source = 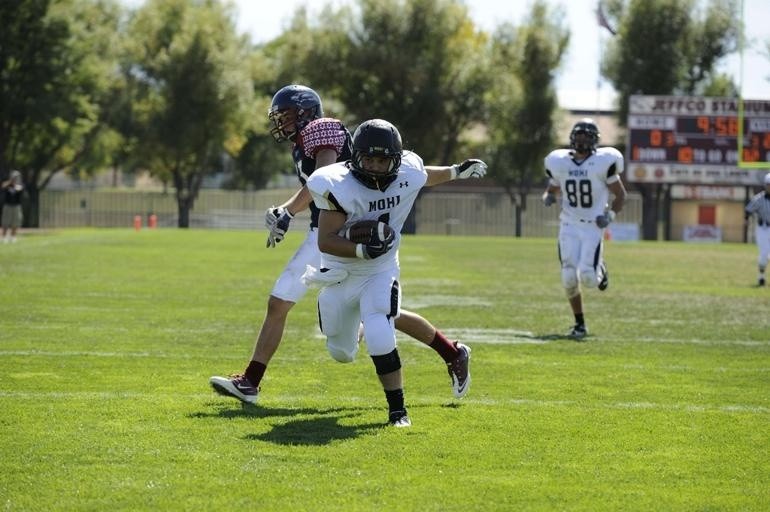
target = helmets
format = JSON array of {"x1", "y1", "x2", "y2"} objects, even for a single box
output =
[
  {"x1": 569, "y1": 118, "x2": 600, "y2": 156},
  {"x1": 345, "y1": 118, "x2": 403, "y2": 190},
  {"x1": 269, "y1": 85, "x2": 323, "y2": 143}
]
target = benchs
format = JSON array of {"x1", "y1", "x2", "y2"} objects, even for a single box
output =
[{"x1": 684, "y1": 225, "x2": 722, "y2": 241}]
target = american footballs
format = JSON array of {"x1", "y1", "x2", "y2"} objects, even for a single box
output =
[{"x1": 344, "y1": 220, "x2": 394, "y2": 245}]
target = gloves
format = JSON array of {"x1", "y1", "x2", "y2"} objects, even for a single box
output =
[
  {"x1": 594, "y1": 211, "x2": 612, "y2": 226},
  {"x1": 265, "y1": 206, "x2": 284, "y2": 227},
  {"x1": 453, "y1": 158, "x2": 488, "y2": 180},
  {"x1": 356, "y1": 241, "x2": 388, "y2": 261},
  {"x1": 541, "y1": 191, "x2": 557, "y2": 208},
  {"x1": 267, "y1": 208, "x2": 294, "y2": 248}
]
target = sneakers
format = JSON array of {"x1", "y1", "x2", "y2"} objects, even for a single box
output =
[
  {"x1": 389, "y1": 407, "x2": 412, "y2": 427},
  {"x1": 446, "y1": 341, "x2": 471, "y2": 399},
  {"x1": 598, "y1": 272, "x2": 608, "y2": 291},
  {"x1": 209, "y1": 375, "x2": 261, "y2": 406}
]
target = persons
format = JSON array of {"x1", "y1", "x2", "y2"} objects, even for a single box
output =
[
  {"x1": 208, "y1": 84, "x2": 472, "y2": 403},
  {"x1": 0, "y1": 170, "x2": 30, "y2": 244},
  {"x1": 540, "y1": 118, "x2": 628, "y2": 337},
  {"x1": 306, "y1": 117, "x2": 489, "y2": 424},
  {"x1": 743, "y1": 173, "x2": 770, "y2": 286}
]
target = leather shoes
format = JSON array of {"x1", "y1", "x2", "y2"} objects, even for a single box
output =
[{"x1": 571, "y1": 324, "x2": 585, "y2": 336}]
